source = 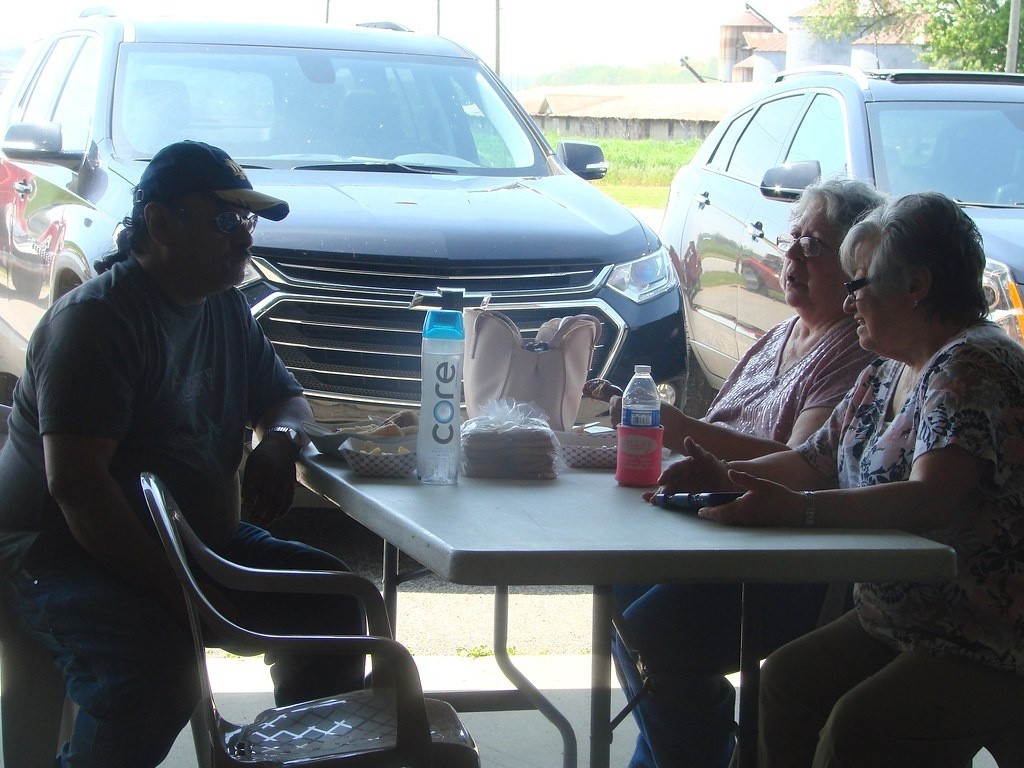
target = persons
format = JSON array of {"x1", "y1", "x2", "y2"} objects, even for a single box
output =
[
  {"x1": 1, "y1": 138, "x2": 368, "y2": 767},
  {"x1": 656, "y1": 189, "x2": 1024, "y2": 768},
  {"x1": 583, "y1": 175, "x2": 890, "y2": 767}
]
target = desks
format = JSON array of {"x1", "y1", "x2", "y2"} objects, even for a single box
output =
[{"x1": 290, "y1": 441, "x2": 959, "y2": 768}]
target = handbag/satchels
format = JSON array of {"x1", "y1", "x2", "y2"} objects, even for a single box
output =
[{"x1": 462, "y1": 303, "x2": 600, "y2": 432}]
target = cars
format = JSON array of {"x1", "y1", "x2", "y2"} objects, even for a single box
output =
[{"x1": 733, "y1": 252, "x2": 785, "y2": 296}]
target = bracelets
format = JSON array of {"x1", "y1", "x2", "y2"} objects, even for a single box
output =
[{"x1": 803, "y1": 490, "x2": 816, "y2": 527}]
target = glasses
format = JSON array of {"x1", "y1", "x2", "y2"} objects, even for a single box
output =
[
  {"x1": 776, "y1": 232, "x2": 837, "y2": 258},
  {"x1": 171, "y1": 205, "x2": 259, "y2": 235},
  {"x1": 842, "y1": 268, "x2": 903, "y2": 297}
]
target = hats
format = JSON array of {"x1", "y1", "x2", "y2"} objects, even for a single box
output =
[{"x1": 135, "y1": 140, "x2": 289, "y2": 221}]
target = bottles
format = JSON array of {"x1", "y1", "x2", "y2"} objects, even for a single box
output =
[
  {"x1": 416, "y1": 310, "x2": 466, "y2": 488},
  {"x1": 618, "y1": 364, "x2": 665, "y2": 487}
]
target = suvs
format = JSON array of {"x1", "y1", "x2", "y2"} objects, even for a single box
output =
[
  {"x1": 0, "y1": 5, "x2": 690, "y2": 508},
  {"x1": 661, "y1": 27, "x2": 1023, "y2": 419}
]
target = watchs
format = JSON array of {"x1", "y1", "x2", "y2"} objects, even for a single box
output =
[{"x1": 261, "y1": 425, "x2": 303, "y2": 460}]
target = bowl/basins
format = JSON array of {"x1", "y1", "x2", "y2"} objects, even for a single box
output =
[{"x1": 302, "y1": 417, "x2": 418, "y2": 461}]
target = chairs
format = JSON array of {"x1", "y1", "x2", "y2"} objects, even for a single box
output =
[{"x1": 137, "y1": 472, "x2": 484, "y2": 768}]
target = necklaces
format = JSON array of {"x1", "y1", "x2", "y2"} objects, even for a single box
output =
[{"x1": 769, "y1": 316, "x2": 843, "y2": 389}]
